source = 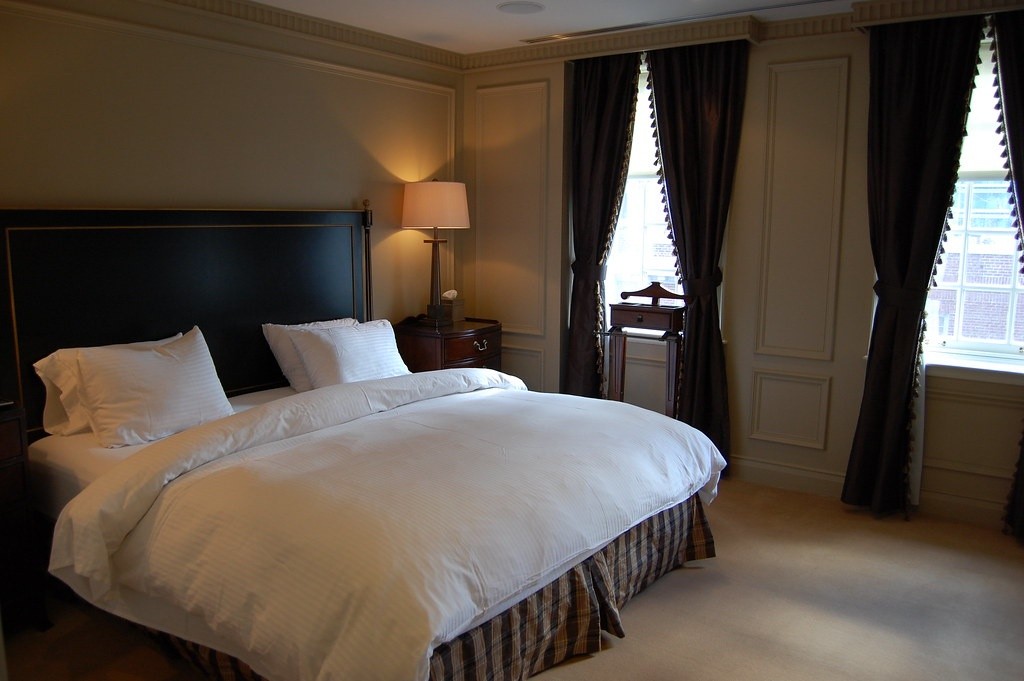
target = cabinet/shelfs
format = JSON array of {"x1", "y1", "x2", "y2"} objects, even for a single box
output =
[{"x1": 595, "y1": 280, "x2": 692, "y2": 419}]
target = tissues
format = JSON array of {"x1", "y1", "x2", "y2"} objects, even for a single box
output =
[{"x1": 440, "y1": 289, "x2": 467, "y2": 323}]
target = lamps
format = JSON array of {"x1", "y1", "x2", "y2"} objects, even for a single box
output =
[{"x1": 400, "y1": 179, "x2": 470, "y2": 328}]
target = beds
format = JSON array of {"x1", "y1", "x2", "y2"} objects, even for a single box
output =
[{"x1": 0, "y1": 198, "x2": 728, "y2": 681}]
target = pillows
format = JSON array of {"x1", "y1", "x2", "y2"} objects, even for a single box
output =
[
  {"x1": 32, "y1": 331, "x2": 182, "y2": 437},
  {"x1": 75, "y1": 323, "x2": 236, "y2": 449},
  {"x1": 287, "y1": 318, "x2": 414, "y2": 388},
  {"x1": 261, "y1": 316, "x2": 359, "y2": 394}
]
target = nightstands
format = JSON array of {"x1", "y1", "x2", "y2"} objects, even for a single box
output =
[
  {"x1": 0, "y1": 406, "x2": 56, "y2": 636},
  {"x1": 393, "y1": 315, "x2": 503, "y2": 374}
]
